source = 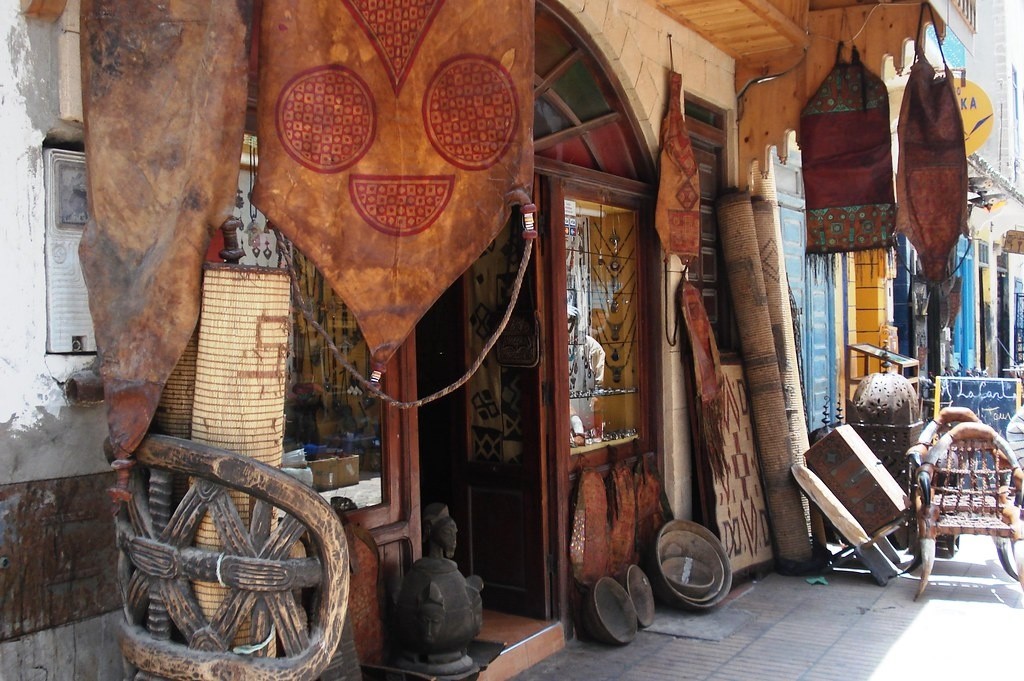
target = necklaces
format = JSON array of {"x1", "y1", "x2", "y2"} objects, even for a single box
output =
[
  {"x1": 236, "y1": 126, "x2": 384, "y2": 481},
  {"x1": 591, "y1": 220, "x2": 636, "y2": 384}
]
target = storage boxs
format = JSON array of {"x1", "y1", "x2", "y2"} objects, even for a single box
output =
[
  {"x1": 802, "y1": 423, "x2": 897, "y2": 535},
  {"x1": 282, "y1": 442, "x2": 360, "y2": 490}
]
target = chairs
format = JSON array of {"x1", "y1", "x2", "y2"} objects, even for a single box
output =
[{"x1": 906, "y1": 406, "x2": 1024, "y2": 602}]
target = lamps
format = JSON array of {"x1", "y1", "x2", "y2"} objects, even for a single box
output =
[{"x1": 912, "y1": 271, "x2": 926, "y2": 315}]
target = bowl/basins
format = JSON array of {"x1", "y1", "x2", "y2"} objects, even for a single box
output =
[
  {"x1": 581, "y1": 576, "x2": 639, "y2": 646},
  {"x1": 662, "y1": 556, "x2": 714, "y2": 599},
  {"x1": 619, "y1": 565, "x2": 655, "y2": 627},
  {"x1": 649, "y1": 519, "x2": 732, "y2": 609},
  {"x1": 660, "y1": 529, "x2": 725, "y2": 604}
]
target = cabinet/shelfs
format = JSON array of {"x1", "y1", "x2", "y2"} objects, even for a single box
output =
[{"x1": 569, "y1": 388, "x2": 639, "y2": 452}]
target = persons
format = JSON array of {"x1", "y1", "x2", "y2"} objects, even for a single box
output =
[
  {"x1": 422, "y1": 502, "x2": 458, "y2": 559},
  {"x1": 415, "y1": 583, "x2": 445, "y2": 646}
]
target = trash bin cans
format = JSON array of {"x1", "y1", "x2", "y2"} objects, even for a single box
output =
[{"x1": 841, "y1": 369, "x2": 926, "y2": 548}]
